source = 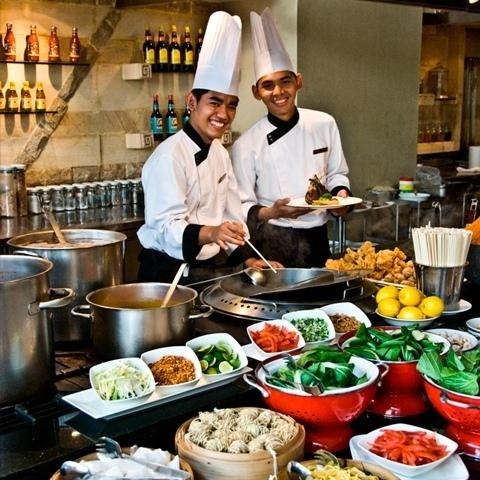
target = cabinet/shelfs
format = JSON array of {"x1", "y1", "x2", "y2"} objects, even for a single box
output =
[{"x1": 417, "y1": 20, "x2": 467, "y2": 154}]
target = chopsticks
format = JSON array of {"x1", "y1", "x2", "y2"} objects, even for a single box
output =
[{"x1": 411, "y1": 221, "x2": 473, "y2": 267}]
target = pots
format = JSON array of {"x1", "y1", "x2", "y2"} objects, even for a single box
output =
[
  {"x1": 7, "y1": 229, "x2": 129, "y2": 348},
  {"x1": 0, "y1": 255, "x2": 76, "y2": 403},
  {"x1": 220, "y1": 265, "x2": 380, "y2": 306},
  {"x1": 70, "y1": 282, "x2": 215, "y2": 362}
]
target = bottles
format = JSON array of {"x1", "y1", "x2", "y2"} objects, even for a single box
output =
[
  {"x1": 2, "y1": 80, "x2": 46, "y2": 112},
  {"x1": 165, "y1": 94, "x2": 178, "y2": 137},
  {"x1": 48, "y1": 26, "x2": 61, "y2": 62},
  {"x1": 26, "y1": 25, "x2": 39, "y2": 61},
  {"x1": 427, "y1": 60, "x2": 449, "y2": 98},
  {"x1": 169, "y1": 24, "x2": 203, "y2": 71},
  {"x1": 0, "y1": 165, "x2": 18, "y2": 217},
  {"x1": 4, "y1": 22, "x2": 17, "y2": 63},
  {"x1": 0, "y1": 203, "x2": 144, "y2": 239},
  {"x1": 11, "y1": 163, "x2": 29, "y2": 217},
  {"x1": 182, "y1": 102, "x2": 191, "y2": 126},
  {"x1": 68, "y1": 28, "x2": 82, "y2": 64},
  {"x1": 149, "y1": 94, "x2": 163, "y2": 140},
  {"x1": 142, "y1": 24, "x2": 156, "y2": 73},
  {"x1": 154, "y1": 25, "x2": 169, "y2": 73},
  {"x1": 27, "y1": 176, "x2": 143, "y2": 214}
]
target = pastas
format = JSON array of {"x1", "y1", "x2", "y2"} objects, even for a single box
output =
[{"x1": 308, "y1": 465, "x2": 378, "y2": 480}]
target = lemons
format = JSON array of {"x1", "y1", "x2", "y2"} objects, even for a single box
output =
[
  {"x1": 396, "y1": 306, "x2": 425, "y2": 320},
  {"x1": 375, "y1": 285, "x2": 398, "y2": 303},
  {"x1": 419, "y1": 296, "x2": 444, "y2": 317},
  {"x1": 377, "y1": 298, "x2": 400, "y2": 317},
  {"x1": 398, "y1": 287, "x2": 423, "y2": 306}
]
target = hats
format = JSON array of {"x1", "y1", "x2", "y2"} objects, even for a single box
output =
[
  {"x1": 191, "y1": 11, "x2": 243, "y2": 100},
  {"x1": 249, "y1": 6, "x2": 294, "y2": 85}
]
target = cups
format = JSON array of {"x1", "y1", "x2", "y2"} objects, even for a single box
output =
[{"x1": 418, "y1": 104, "x2": 457, "y2": 143}]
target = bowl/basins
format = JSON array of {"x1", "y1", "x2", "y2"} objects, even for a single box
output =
[
  {"x1": 338, "y1": 327, "x2": 450, "y2": 419},
  {"x1": 421, "y1": 357, "x2": 480, "y2": 458},
  {"x1": 246, "y1": 352, "x2": 390, "y2": 454}
]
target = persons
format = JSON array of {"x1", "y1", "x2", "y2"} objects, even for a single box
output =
[
  {"x1": 230, "y1": 71, "x2": 356, "y2": 268},
  {"x1": 134, "y1": 88, "x2": 286, "y2": 290}
]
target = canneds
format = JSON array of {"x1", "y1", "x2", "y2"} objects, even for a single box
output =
[{"x1": 26, "y1": 178, "x2": 145, "y2": 213}]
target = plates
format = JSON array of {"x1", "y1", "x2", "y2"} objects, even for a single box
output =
[
  {"x1": 185, "y1": 334, "x2": 246, "y2": 378},
  {"x1": 285, "y1": 194, "x2": 363, "y2": 210},
  {"x1": 246, "y1": 320, "x2": 304, "y2": 357},
  {"x1": 63, "y1": 367, "x2": 253, "y2": 420},
  {"x1": 90, "y1": 358, "x2": 156, "y2": 403},
  {"x1": 281, "y1": 310, "x2": 337, "y2": 347},
  {"x1": 466, "y1": 317, "x2": 479, "y2": 335},
  {"x1": 398, "y1": 192, "x2": 431, "y2": 202},
  {"x1": 242, "y1": 342, "x2": 272, "y2": 361},
  {"x1": 140, "y1": 345, "x2": 202, "y2": 396},
  {"x1": 350, "y1": 434, "x2": 470, "y2": 478},
  {"x1": 425, "y1": 328, "x2": 479, "y2": 352},
  {"x1": 371, "y1": 201, "x2": 395, "y2": 209},
  {"x1": 352, "y1": 204, "x2": 371, "y2": 213},
  {"x1": 359, "y1": 424, "x2": 459, "y2": 474},
  {"x1": 319, "y1": 301, "x2": 372, "y2": 333},
  {"x1": 375, "y1": 307, "x2": 440, "y2": 326}
]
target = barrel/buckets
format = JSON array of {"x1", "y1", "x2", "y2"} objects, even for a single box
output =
[{"x1": 414, "y1": 261, "x2": 470, "y2": 312}]
